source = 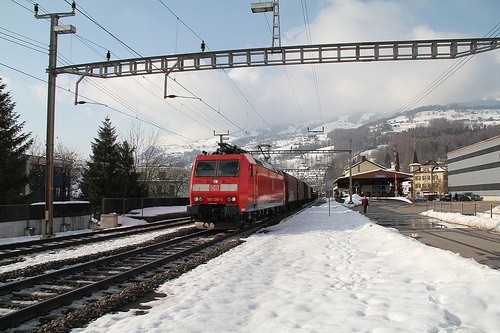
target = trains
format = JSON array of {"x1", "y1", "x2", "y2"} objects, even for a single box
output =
[{"x1": 187, "y1": 143, "x2": 318, "y2": 230}]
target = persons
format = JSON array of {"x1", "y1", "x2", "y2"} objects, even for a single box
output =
[
  {"x1": 362, "y1": 196, "x2": 369, "y2": 213},
  {"x1": 449, "y1": 193, "x2": 452, "y2": 201},
  {"x1": 455, "y1": 193, "x2": 459, "y2": 201}
]
